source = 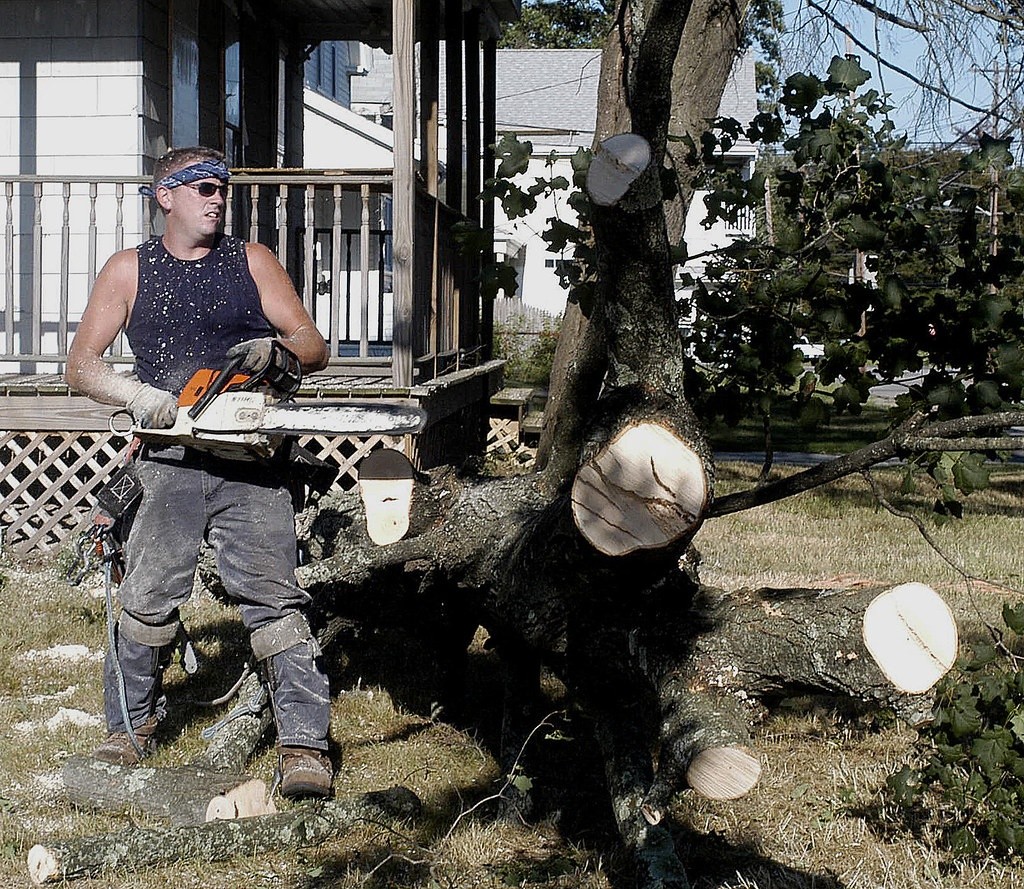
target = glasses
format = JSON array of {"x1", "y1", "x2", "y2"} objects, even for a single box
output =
[{"x1": 168, "y1": 177, "x2": 228, "y2": 200}]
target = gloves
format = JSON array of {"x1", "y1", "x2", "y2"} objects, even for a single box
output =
[
  {"x1": 127, "y1": 382, "x2": 178, "y2": 429},
  {"x1": 226, "y1": 337, "x2": 290, "y2": 382}
]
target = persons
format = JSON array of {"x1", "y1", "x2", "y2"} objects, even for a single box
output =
[{"x1": 62, "y1": 146, "x2": 335, "y2": 797}]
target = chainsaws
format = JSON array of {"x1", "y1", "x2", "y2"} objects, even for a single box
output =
[{"x1": 129, "y1": 338, "x2": 428, "y2": 461}]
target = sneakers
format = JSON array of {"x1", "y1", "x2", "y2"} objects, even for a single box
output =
[
  {"x1": 93, "y1": 714, "x2": 159, "y2": 765},
  {"x1": 276, "y1": 746, "x2": 334, "y2": 798}
]
